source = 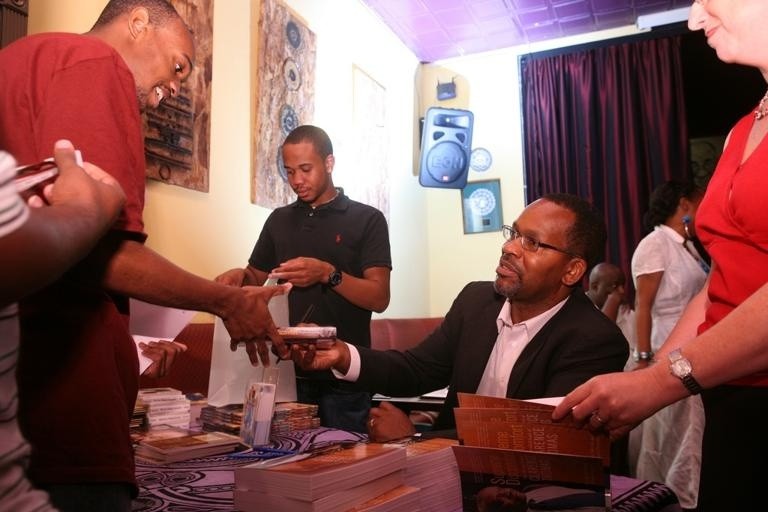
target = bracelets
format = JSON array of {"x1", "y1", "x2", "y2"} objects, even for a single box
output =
[
  {"x1": 631, "y1": 350, "x2": 654, "y2": 363},
  {"x1": 668, "y1": 348, "x2": 704, "y2": 396}
]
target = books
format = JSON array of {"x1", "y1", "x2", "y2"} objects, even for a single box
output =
[
  {"x1": 447, "y1": 380, "x2": 611, "y2": 509},
  {"x1": 232, "y1": 436, "x2": 457, "y2": 512},
  {"x1": 131, "y1": 380, "x2": 322, "y2": 466}
]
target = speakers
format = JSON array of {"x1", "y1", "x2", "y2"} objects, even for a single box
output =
[{"x1": 419, "y1": 106, "x2": 473, "y2": 189}]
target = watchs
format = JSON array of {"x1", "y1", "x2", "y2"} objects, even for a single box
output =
[{"x1": 325, "y1": 267, "x2": 344, "y2": 288}]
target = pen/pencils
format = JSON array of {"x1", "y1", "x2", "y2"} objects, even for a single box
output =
[
  {"x1": 253, "y1": 445, "x2": 307, "y2": 454},
  {"x1": 227, "y1": 452, "x2": 284, "y2": 459},
  {"x1": 276, "y1": 304, "x2": 316, "y2": 364},
  {"x1": 158, "y1": 350, "x2": 167, "y2": 378}
]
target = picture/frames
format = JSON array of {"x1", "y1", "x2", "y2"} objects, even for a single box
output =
[{"x1": 461, "y1": 179, "x2": 503, "y2": 234}]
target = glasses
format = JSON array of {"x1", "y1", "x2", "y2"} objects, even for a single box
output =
[{"x1": 501, "y1": 224, "x2": 583, "y2": 259}]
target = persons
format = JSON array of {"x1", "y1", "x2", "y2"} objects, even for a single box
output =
[
  {"x1": 199, "y1": 123, "x2": 396, "y2": 441},
  {"x1": 582, "y1": 260, "x2": 638, "y2": 347},
  {"x1": 0, "y1": 138, "x2": 127, "y2": 512},
  {"x1": 0, "y1": 0, "x2": 296, "y2": 510},
  {"x1": 613, "y1": 176, "x2": 722, "y2": 511},
  {"x1": 551, "y1": 0, "x2": 768, "y2": 510},
  {"x1": 273, "y1": 193, "x2": 632, "y2": 509}
]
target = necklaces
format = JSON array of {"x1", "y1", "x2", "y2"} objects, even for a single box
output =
[{"x1": 754, "y1": 90, "x2": 768, "y2": 120}]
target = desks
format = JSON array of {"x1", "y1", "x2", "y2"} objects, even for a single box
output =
[{"x1": 129, "y1": 438, "x2": 683, "y2": 512}]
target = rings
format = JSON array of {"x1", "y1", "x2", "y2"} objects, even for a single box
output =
[
  {"x1": 370, "y1": 417, "x2": 376, "y2": 427},
  {"x1": 591, "y1": 415, "x2": 607, "y2": 427}
]
